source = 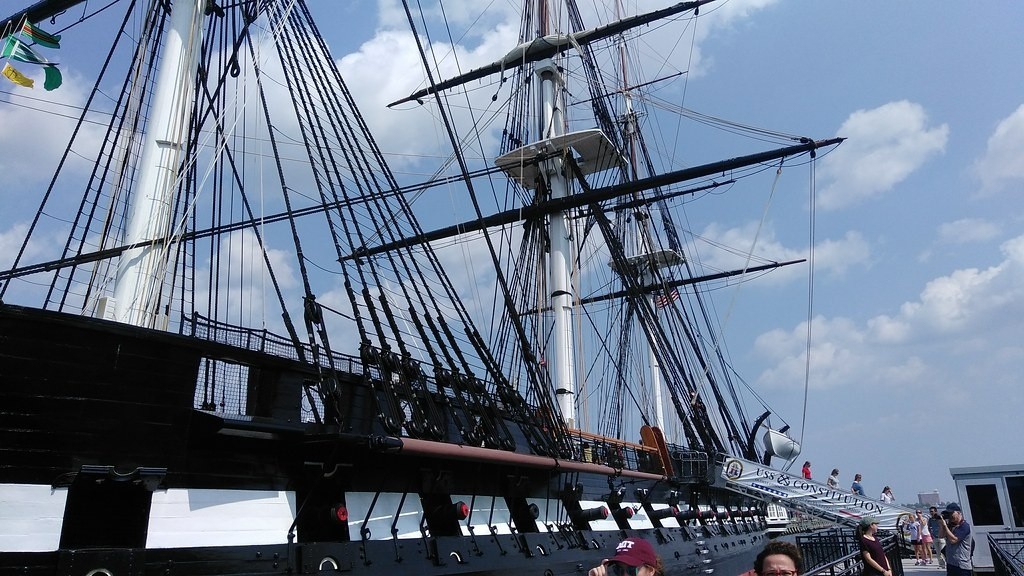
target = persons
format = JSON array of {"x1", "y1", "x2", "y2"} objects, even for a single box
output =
[
  {"x1": 826, "y1": 468, "x2": 840, "y2": 489},
  {"x1": 851, "y1": 473, "x2": 865, "y2": 496},
  {"x1": 589, "y1": 537, "x2": 664, "y2": 576},
  {"x1": 801, "y1": 461, "x2": 813, "y2": 480},
  {"x1": 754, "y1": 539, "x2": 805, "y2": 576},
  {"x1": 902, "y1": 502, "x2": 973, "y2": 576},
  {"x1": 855, "y1": 515, "x2": 892, "y2": 576},
  {"x1": 880, "y1": 486, "x2": 896, "y2": 504}
]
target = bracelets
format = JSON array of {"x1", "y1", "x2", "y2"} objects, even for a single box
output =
[
  {"x1": 942, "y1": 524, "x2": 947, "y2": 527},
  {"x1": 887, "y1": 568, "x2": 892, "y2": 570},
  {"x1": 881, "y1": 569, "x2": 886, "y2": 574}
]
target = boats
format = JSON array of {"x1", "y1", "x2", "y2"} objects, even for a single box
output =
[{"x1": 762, "y1": 428, "x2": 802, "y2": 461}]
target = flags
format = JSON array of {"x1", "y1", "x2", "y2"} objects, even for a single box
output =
[{"x1": 1, "y1": 19, "x2": 62, "y2": 91}]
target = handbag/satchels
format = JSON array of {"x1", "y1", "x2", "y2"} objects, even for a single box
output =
[{"x1": 940, "y1": 537, "x2": 975, "y2": 558}]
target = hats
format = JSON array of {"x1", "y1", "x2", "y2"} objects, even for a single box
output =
[
  {"x1": 941, "y1": 502, "x2": 961, "y2": 513},
  {"x1": 860, "y1": 517, "x2": 880, "y2": 527},
  {"x1": 602, "y1": 537, "x2": 656, "y2": 567}
]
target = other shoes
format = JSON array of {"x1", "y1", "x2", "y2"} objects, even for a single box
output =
[
  {"x1": 928, "y1": 560, "x2": 933, "y2": 563},
  {"x1": 915, "y1": 560, "x2": 920, "y2": 565},
  {"x1": 919, "y1": 560, "x2": 927, "y2": 566}
]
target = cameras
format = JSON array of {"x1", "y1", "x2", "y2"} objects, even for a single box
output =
[{"x1": 934, "y1": 510, "x2": 951, "y2": 520}]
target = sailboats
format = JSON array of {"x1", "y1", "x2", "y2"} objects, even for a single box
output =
[{"x1": 0, "y1": 1, "x2": 852, "y2": 576}]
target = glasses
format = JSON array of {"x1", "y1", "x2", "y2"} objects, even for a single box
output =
[
  {"x1": 857, "y1": 477, "x2": 861, "y2": 478},
  {"x1": 836, "y1": 473, "x2": 838, "y2": 474},
  {"x1": 917, "y1": 512, "x2": 922, "y2": 513},
  {"x1": 930, "y1": 511, "x2": 935, "y2": 512},
  {"x1": 606, "y1": 563, "x2": 646, "y2": 576},
  {"x1": 760, "y1": 570, "x2": 797, "y2": 576}
]
